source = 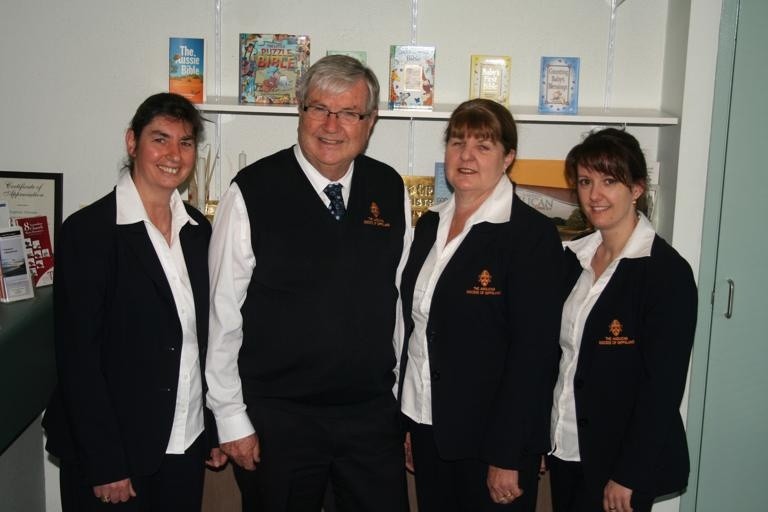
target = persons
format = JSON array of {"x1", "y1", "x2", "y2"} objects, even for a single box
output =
[
  {"x1": 41, "y1": 92, "x2": 212, "y2": 511},
  {"x1": 544, "y1": 127, "x2": 698, "y2": 512},
  {"x1": 399, "y1": 99, "x2": 565, "y2": 511},
  {"x1": 204, "y1": 55, "x2": 412, "y2": 512}
]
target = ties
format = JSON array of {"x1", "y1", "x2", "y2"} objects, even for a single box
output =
[{"x1": 325, "y1": 182, "x2": 345, "y2": 222}]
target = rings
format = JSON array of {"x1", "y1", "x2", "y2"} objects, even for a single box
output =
[
  {"x1": 499, "y1": 497, "x2": 505, "y2": 503},
  {"x1": 609, "y1": 507, "x2": 616, "y2": 510},
  {"x1": 506, "y1": 492, "x2": 512, "y2": 498}
]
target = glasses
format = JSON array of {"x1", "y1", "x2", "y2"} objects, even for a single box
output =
[{"x1": 301, "y1": 101, "x2": 372, "y2": 126}]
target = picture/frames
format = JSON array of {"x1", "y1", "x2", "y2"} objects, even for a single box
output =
[{"x1": 0, "y1": 172, "x2": 63, "y2": 292}]
